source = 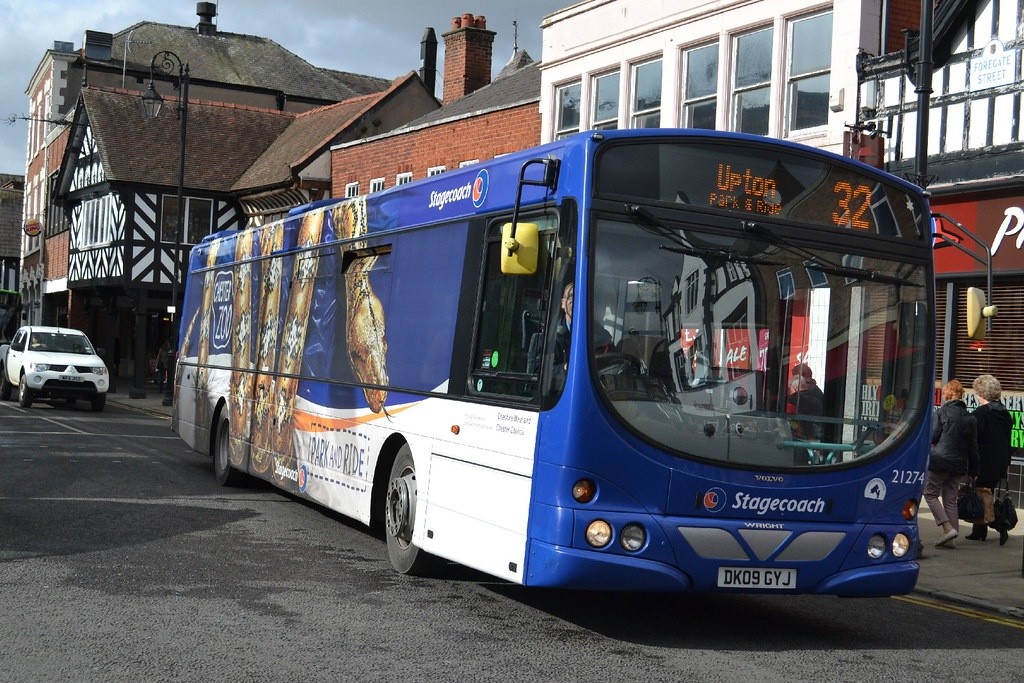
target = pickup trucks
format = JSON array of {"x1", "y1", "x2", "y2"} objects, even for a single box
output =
[{"x1": 0, "y1": 326, "x2": 109, "y2": 412}]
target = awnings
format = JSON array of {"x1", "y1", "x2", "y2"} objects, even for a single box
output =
[{"x1": 0, "y1": 288, "x2": 22, "y2": 297}]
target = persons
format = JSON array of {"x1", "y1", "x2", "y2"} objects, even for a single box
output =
[
  {"x1": 923, "y1": 375, "x2": 1013, "y2": 549},
  {"x1": 156, "y1": 340, "x2": 171, "y2": 394},
  {"x1": 530, "y1": 281, "x2": 617, "y2": 402},
  {"x1": 786, "y1": 363, "x2": 823, "y2": 466}
]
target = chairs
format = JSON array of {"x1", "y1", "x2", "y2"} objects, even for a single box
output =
[{"x1": 525, "y1": 332, "x2": 544, "y2": 377}]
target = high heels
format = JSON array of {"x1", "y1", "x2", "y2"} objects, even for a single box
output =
[
  {"x1": 999, "y1": 531, "x2": 1008, "y2": 545},
  {"x1": 964, "y1": 531, "x2": 988, "y2": 542}
]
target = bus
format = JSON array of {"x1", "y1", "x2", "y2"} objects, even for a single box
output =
[
  {"x1": 0, "y1": 289, "x2": 29, "y2": 344},
  {"x1": 171, "y1": 127, "x2": 998, "y2": 600}
]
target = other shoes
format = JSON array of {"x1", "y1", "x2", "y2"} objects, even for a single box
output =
[
  {"x1": 934, "y1": 529, "x2": 958, "y2": 546},
  {"x1": 942, "y1": 538, "x2": 957, "y2": 548}
]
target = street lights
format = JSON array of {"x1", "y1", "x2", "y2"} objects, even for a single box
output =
[{"x1": 141, "y1": 51, "x2": 191, "y2": 406}]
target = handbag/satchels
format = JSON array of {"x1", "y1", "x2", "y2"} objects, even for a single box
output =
[
  {"x1": 988, "y1": 476, "x2": 1019, "y2": 530},
  {"x1": 965, "y1": 488, "x2": 995, "y2": 524},
  {"x1": 957, "y1": 476, "x2": 986, "y2": 522}
]
